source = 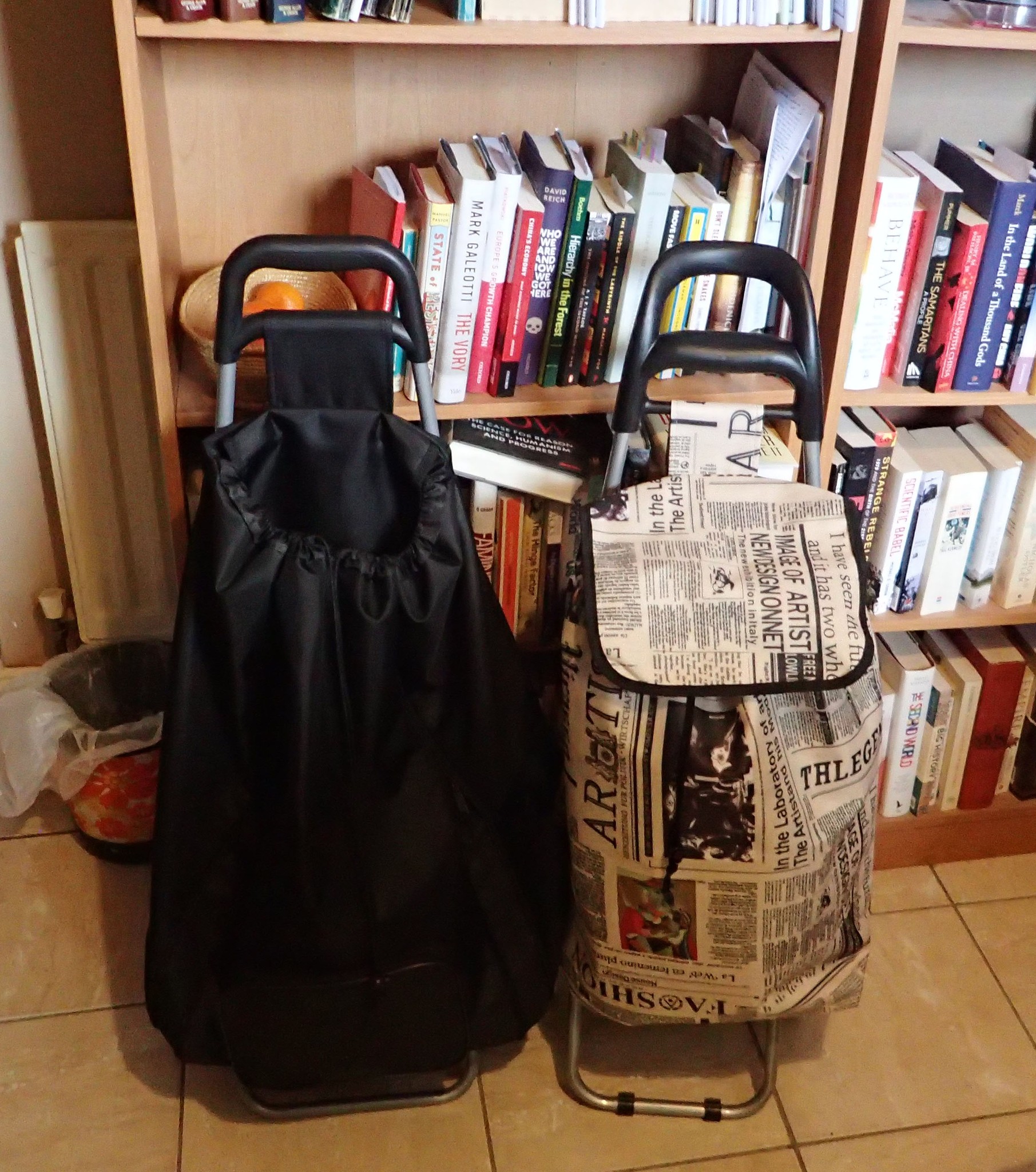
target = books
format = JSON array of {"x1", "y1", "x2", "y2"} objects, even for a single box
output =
[{"x1": 156, "y1": 0, "x2": 1036, "y2": 817}]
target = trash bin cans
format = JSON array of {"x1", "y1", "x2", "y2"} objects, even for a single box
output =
[{"x1": 42, "y1": 636, "x2": 172, "y2": 865}]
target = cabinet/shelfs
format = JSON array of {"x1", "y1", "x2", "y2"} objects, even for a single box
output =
[{"x1": 112, "y1": 0, "x2": 1036, "y2": 872}]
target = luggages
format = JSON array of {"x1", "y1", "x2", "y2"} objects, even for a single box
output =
[
  {"x1": 558, "y1": 242, "x2": 883, "y2": 1119},
  {"x1": 142, "y1": 235, "x2": 556, "y2": 1118}
]
